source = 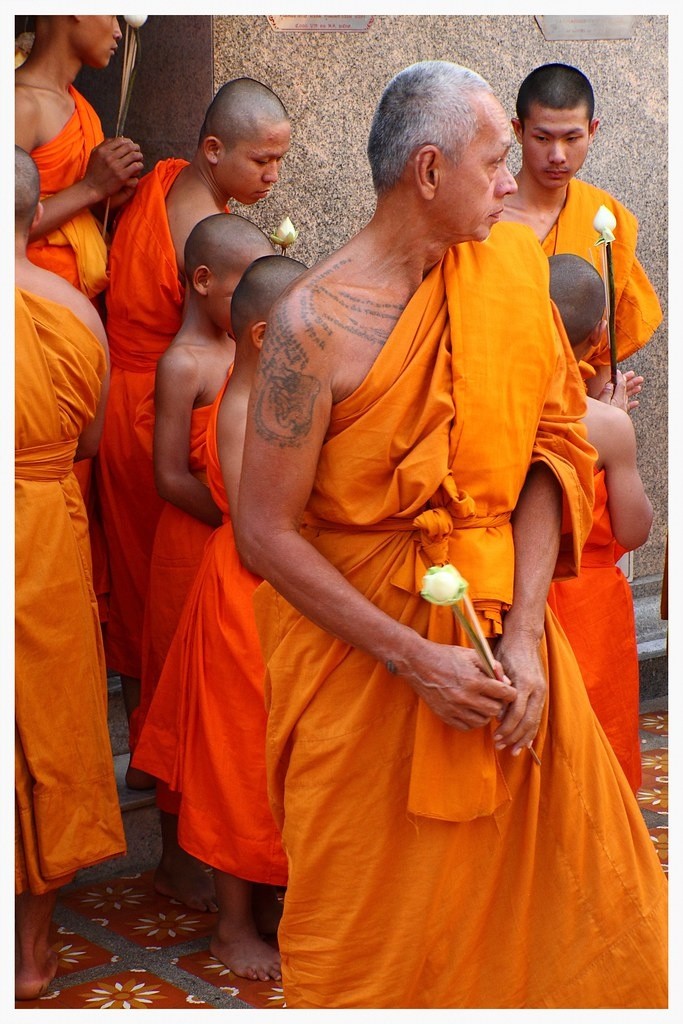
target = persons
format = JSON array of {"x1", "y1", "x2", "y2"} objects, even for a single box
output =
[
  {"x1": 15, "y1": 15, "x2": 143, "y2": 624},
  {"x1": 548, "y1": 252, "x2": 653, "y2": 798},
  {"x1": 236, "y1": 59, "x2": 669, "y2": 1010},
  {"x1": 129, "y1": 254, "x2": 310, "y2": 979},
  {"x1": 102, "y1": 79, "x2": 290, "y2": 787},
  {"x1": 497, "y1": 63, "x2": 663, "y2": 400},
  {"x1": 15, "y1": 146, "x2": 127, "y2": 999},
  {"x1": 137, "y1": 212, "x2": 276, "y2": 916}
]
